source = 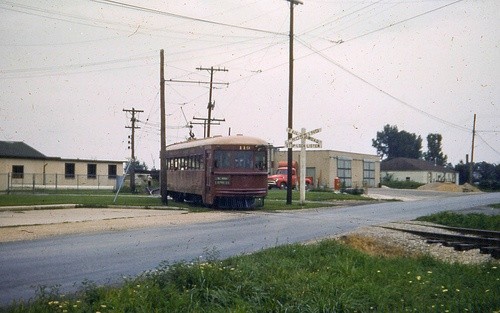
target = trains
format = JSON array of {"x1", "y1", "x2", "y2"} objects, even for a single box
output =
[{"x1": 159, "y1": 133, "x2": 272, "y2": 210}]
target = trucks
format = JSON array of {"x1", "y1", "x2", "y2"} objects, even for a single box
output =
[{"x1": 267, "y1": 160, "x2": 313, "y2": 189}]
